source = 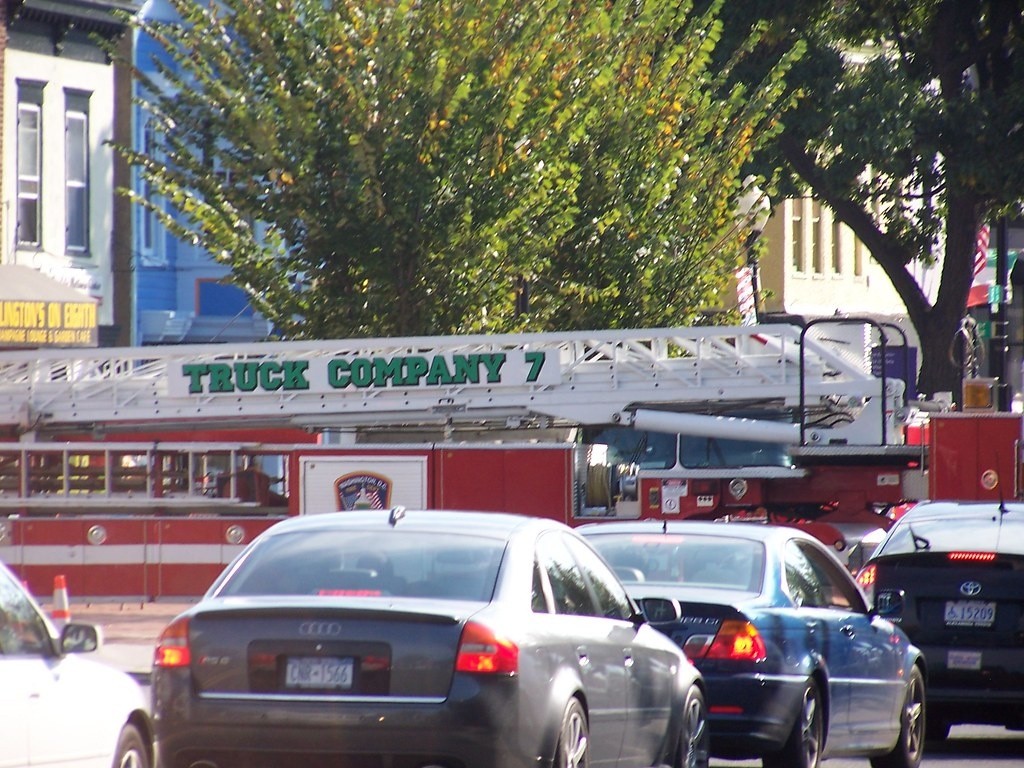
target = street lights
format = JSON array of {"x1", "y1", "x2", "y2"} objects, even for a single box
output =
[{"x1": 731, "y1": 173, "x2": 770, "y2": 323}]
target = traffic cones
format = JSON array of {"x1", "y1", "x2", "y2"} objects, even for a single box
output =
[{"x1": 51, "y1": 574, "x2": 70, "y2": 635}]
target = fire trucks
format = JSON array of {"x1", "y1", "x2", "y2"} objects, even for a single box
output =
[{"x1": 0, "y1": 308, "x2": 1024, "y2": 603}]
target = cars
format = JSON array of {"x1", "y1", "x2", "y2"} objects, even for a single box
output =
[
  {"x1": 564, "y1": 519, "x2": 928, "y2": 768},
  {"x1": 1, "y1": 560, "x2": 154, "y2": 767},
  {"x1": 852, "y1": 498, "x2": 1024, "y2": 749},
  {"x1": 149, "y1": 505, "x2": 714, "y2": 768}
]
